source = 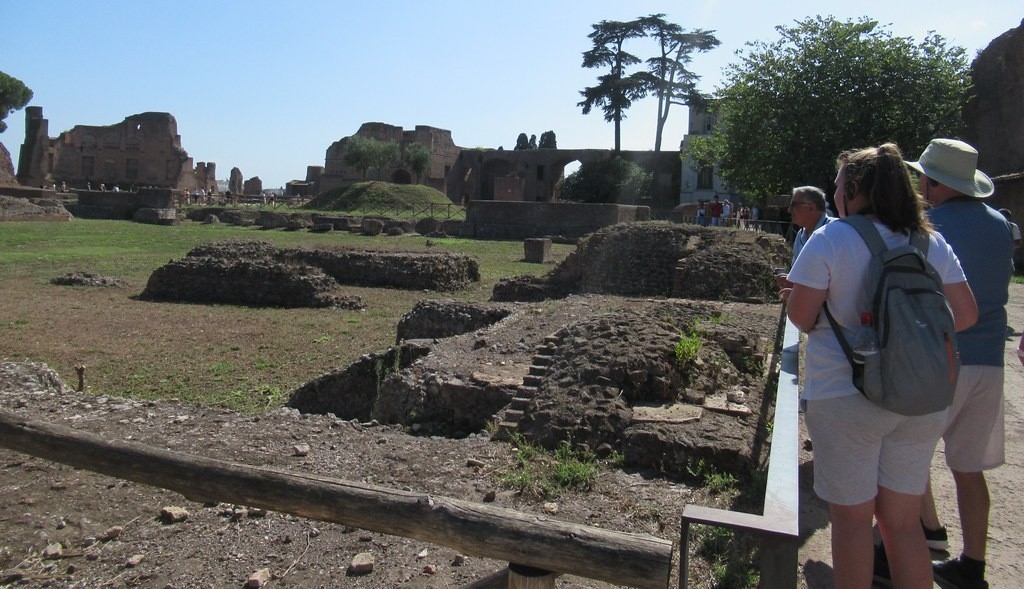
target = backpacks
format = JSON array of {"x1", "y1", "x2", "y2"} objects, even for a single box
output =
[{"x1": 822, "y1": 211, "x2": 961, "y2": 417}]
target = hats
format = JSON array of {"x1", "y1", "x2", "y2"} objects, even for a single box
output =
[{"x1": 902, "y1": 137, "x2": 994, "y2": 200}]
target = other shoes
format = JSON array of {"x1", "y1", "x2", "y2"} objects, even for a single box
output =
[
  {"x1": 873, "y1": 539, "x2": 891, "y2": 584},
  {"x1": 931, "y1": 557, "x2": 990, "y2": 589},
  {"x1": 918, "y1": 516, "x2": 949, "y2": 549}
]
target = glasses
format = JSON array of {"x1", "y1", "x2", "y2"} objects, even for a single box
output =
[{"x1": 790, "y1": 200, "x2": 816, "y2": 209}]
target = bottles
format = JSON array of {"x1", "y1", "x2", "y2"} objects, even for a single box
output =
[{"x1": 852, "y1": 311, "x2": 879, "y2": 390}]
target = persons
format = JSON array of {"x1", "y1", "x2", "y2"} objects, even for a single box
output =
[
  {"x1": 60, "y1": 181, "x2": 66, "y2": 193},
  {"x1": 261, "y1": 191, "x2": 277, "y2": 206},
  {"x1": 789, "y1": 186, "x2": 839, "y2": 452},
  {"x1": 786, "y1": 141, "x2": 978, "y2": 589},
  {"x1": 874, "y1": 138, "x2": 1015, "y2": 589},
  {"x1": 997, "y1": 208, "x2": 1022, "y2": 274},
  {"x1": 112, "y1": 185, "x2": 120, "y2": 192},
  {"x1": 184, "y1": 185, "x2": 215, "y2": 206},
  {"x1": 87, "y1": 181, "x2": 91, "y2": 191},
  {"x1": 100, "y1": 183, "x2": 106, "y2": 191},
  {"x1": 225, "y1": 189, "x2": 231, "y2": 205},
  {"x1": 50, "y1": 182, "x2": 56, "y2": 190},
  {"x1": 681, "y1": 184, "x2": 797, "y2": 246}
]
